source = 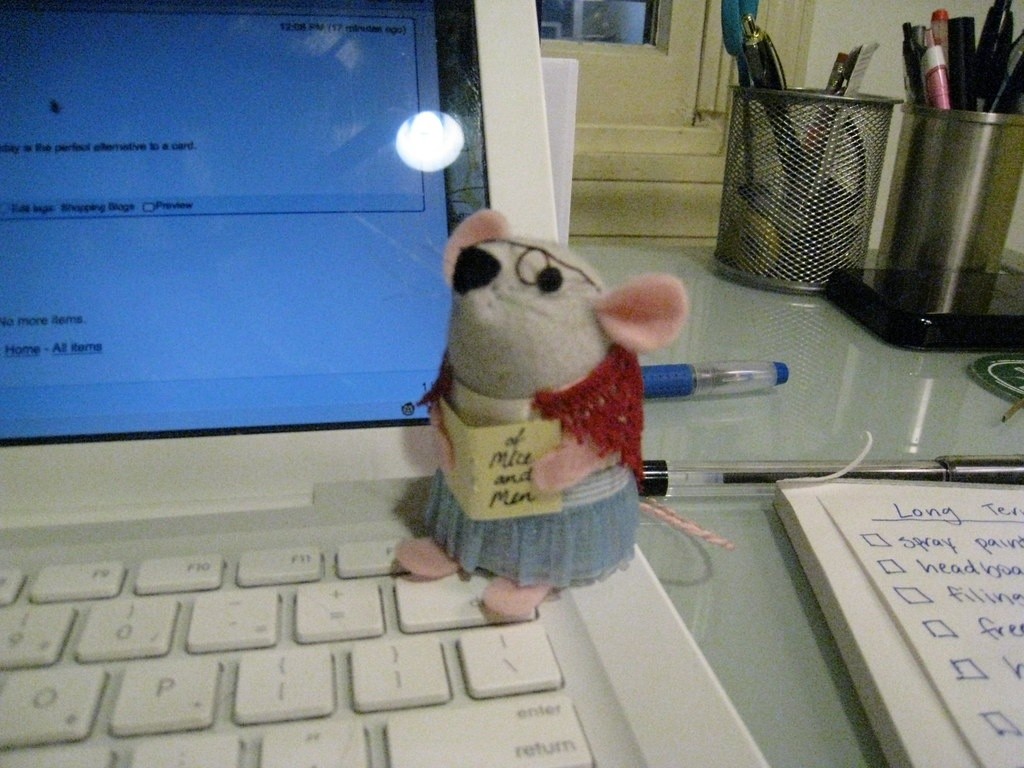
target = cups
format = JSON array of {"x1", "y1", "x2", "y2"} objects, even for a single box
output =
[{"x1": 875, "y1": 106, "x2": 1024, "y2": 272}]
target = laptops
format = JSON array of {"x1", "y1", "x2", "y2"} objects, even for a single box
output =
[{"x1": 0, "y1": 0, "x2": 770, "y2": 768}]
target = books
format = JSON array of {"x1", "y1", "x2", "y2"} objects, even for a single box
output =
[{"x1": 773, "y1": 480, "x2": 1024, "y2": 768}]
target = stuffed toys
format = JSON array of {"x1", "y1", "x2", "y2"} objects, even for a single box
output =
[{"x1": 393, "y1": 208, "x2": 688, "y2": 615}]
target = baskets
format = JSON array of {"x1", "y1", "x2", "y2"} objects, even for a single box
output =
[{"x1": 713, "y1": 84, "x2": 904, "y2": 296}]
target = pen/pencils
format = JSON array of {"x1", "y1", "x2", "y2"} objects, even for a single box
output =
[
  {"x1": 640, "y1": 360, "x2": 790, "y2": 396},
  {"x1": 739, "y1": 12, "x2": 878, "y2": 221},
  {"x1": 902, "y1": 0, "x2": 1024, "y2": 119},
  {"x1": 635, "y1": 454, "x2": 1024, "y2": 495}
]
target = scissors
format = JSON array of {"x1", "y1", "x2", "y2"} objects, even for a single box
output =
[{"x1": 717, "y1": 0, "x2": 763, "y2": 183}]
